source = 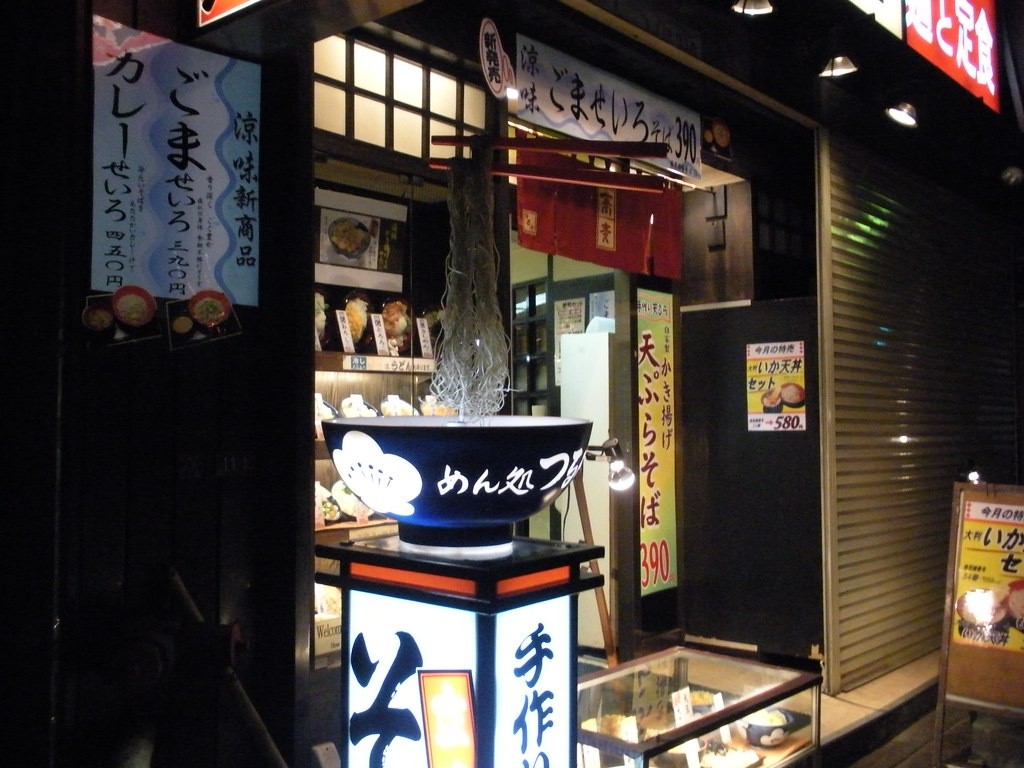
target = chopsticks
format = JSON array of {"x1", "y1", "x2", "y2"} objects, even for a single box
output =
[{"x1": 428, "y1": 134, "x2": 669, "y2": 196}]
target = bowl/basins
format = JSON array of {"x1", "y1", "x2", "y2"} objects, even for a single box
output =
[
  {"x1": 324, "y1": 415, "x2": 592, "y2": 547},
  {"x1": 316, "y1": 289, "x2": 458, "y2": 520},
  {"x1": 189, "y1": 290, "x2": 230, "y2": 328},
  {"x1": 111, "y1": 285, "x2": 156, "y2": 327},
  {"x1": 761, "y1": 390, "x2": 782, "y2": 413},
  {"x1": 580, "y1": 689, "x2": 793, "y2": 768},
  {"x1": 1002, "y1": 579, "x2": 1024, "y2": 631},
  {"x1": 171, "y1": 316, "x2": 193, "y2": 335},
  {"x1": 957, "y1": 589, "x2": 1007, "y2": 627},
  {"x1": 779, "y1": 383, "x2": 805, "y2": 408},
  {"x1": 82, "y1": 305, "x2": 113, "y2": 332},
  {"x1": 328, "y1": 217, "x2": 371, "y2": 258}
]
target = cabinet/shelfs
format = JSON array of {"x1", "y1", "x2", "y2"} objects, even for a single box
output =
[{"x1": 315, "y1": 351, "x2": 436, "y2": 545}]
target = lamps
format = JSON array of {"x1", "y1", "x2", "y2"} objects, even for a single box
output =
[{"x1": 586, "y1": 437, "x2": 635, "y2": 490}]
